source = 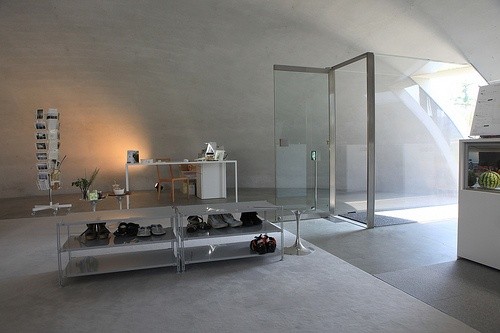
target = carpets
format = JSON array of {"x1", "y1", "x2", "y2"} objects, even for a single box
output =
[{"x1": 338, "y1": 210, "x2": 418, "y2": 227}]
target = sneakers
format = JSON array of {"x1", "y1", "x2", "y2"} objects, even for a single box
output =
[
  {"x1": 263, "y1": 235, "x2": 277, "y2": 253},
  {"x1": 250, "y1": 233, "x2": 267, "y2": 255}
]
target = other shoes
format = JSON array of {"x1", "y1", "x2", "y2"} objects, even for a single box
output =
[
  {"x1": 186, "y1": 215, "x2": 209, "y2": 233},
  {"x1": 150, "y1": 224, "x2": 167, "y2": 237},
  {"x1": 96, "y1": 222, "x2": 111, "y2": 239},
  {"x1": 80, "y1": 223, "x2": 98, "y2": 240},
  {"x1": 112, "y1": 222, "x2": 140, "y2": 238},
  {"x1": 207, "y1": 214, "x2": 228, "y2": 229},
  {"x1": 240, "y1": 211, "x2": 262, "y2": 227},
  {"x1": 219, "y1": 214, "x2": 242, "y2": 228},
  {"x1": 137, "y1": 226, "x2": 151, "y2": 237}
]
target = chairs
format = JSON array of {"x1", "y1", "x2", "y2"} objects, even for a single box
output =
[{"x1": 156, "y1": 158, "x2": 190, "y2": 201}]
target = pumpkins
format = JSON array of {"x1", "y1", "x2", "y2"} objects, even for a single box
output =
[{"x1": 478, "y1": 168, "x2": 500, "y2": 188}]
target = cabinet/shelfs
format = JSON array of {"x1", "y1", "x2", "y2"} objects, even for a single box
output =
[
  {"x1": 53, "y1": 207, "x2": 182, "y2": 290},
  {"x1": 455, "y1": 136, "x2": 500, "y2": 272},
  {"x1": 180, "y1": 198, "x2": 290, "y2": 276}
]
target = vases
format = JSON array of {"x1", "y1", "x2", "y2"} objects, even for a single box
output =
[{"x1": 82, "y1": 190, "x2": 89, "y2": 199}]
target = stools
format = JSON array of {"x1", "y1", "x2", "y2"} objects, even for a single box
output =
[{"x1": 282, "y1": 202, "x2": 314, "y2": 258}]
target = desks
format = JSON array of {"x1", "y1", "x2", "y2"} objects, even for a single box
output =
[
  {"x1": 109, "y1": 189, "x2": 133, "y2": 209},
  {"x1": 124, "y1": 160, "x2": 238, "y2": 204},
  {"x1": 79, "y1": 195, "x2": 106, "y2": 209}
]
